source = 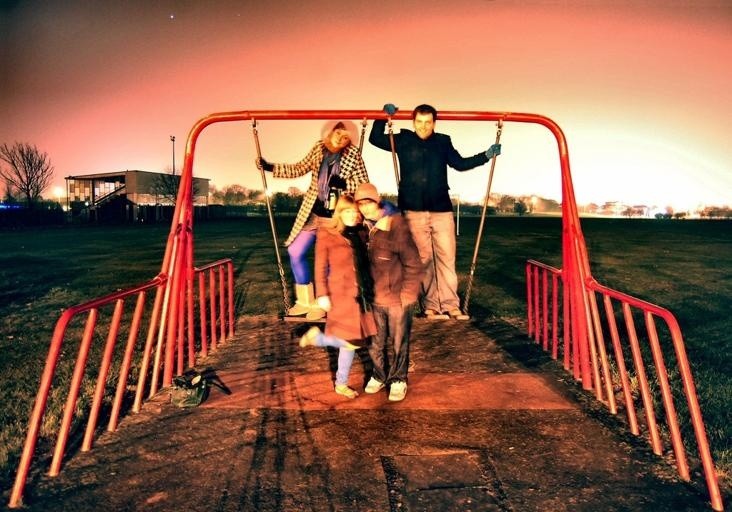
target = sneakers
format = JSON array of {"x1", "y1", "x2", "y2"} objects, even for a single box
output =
[
  {"x1": 334, "y1": 385, "x2": 357, "y2": 397},
  {"x1": 299, "y1": 327, "x2": 320, "y2": 348},
  {"x1": 388, "y1": 381, "x2": 408, "y2": 402},
  {"x1": 365, "y1": 376, "x2": 386, "y2": 394}
]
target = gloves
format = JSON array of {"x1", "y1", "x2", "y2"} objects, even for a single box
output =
[
  {"x1": 485, "y1": 144, "x2": 501, "y2": 158},
  {"x1": 384, "y1": 104, "x2": 399, "y2": 114}
]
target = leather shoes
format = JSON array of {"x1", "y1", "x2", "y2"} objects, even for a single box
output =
[
  {"x1": 449, "y1": 308, "x2": 462, "y2": 317},
  {"x1": 424, "y1": 309, "x2": 436, "y2": 317}
]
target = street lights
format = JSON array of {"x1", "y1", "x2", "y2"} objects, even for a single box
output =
[{"x1": 169, "y1": 135, "x2": 177, "y2": 175}]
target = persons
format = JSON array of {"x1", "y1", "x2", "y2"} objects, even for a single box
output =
[
  {"x1": 298, "y1": 193, "x2": 376, "y2": 401},
  {"x1": 353, "y1": 182, "x2": 421, "y2": 402},
  {"x1": 369, "y1": 103, "x2": 502, "y2": 318}
]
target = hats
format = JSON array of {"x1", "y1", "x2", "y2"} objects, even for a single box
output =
[{"x1": 355, "y1": 183, "x2": 380, "y2": 203}]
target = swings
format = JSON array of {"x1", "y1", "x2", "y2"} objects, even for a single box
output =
[{"x1": 387, "y1": 119, "x2": 503, "y2": 321}]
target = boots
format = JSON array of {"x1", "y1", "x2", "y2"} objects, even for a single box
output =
[{"x1": 287, "y1": 281, "x2": 326, "y2": 320}]
